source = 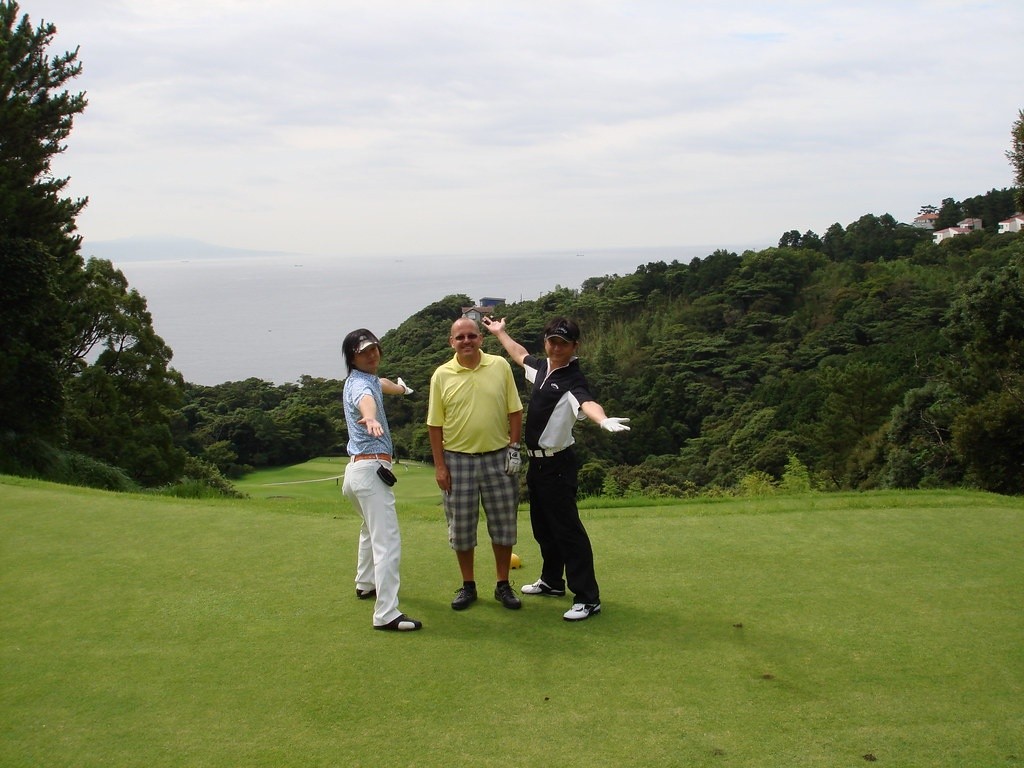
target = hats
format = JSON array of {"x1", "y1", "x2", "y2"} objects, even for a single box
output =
[
  {"x1": 545, "y1": 326, "x2": 576, "y2": 343},
  {"x1": 352, "y1": 334, "x2": 379, "y2": 354}
]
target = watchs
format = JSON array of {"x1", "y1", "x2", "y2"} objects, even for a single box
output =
[{"x1": 508, "y1": 441, "x2": 521, "y2": 450}]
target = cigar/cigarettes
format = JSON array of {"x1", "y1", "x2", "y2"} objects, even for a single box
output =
[{"x1": 446, "y1": 489, "x2": 449, "y2": 496}]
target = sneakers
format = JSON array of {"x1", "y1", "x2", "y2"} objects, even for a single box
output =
[
  {"x1": 563, "y1": 602, "x2": 601, "y2": 620},
  {"x1": 373, "y1": 614, "x2": 422, "y2": 630},
  {"x1": 356, "y1": 589, "x2": 376, "y2": 599},
  {"x1": 521, "y1": 579, "x2": 566, "y2": 596}
]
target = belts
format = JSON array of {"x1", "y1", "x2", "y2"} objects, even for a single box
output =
[
  {"x1": 526, "y1": 437, "x2": 575, "y2": 457},
  {"x1": 444, "y1": 446, "x2": 507, "y2": 455},
  {"x1": 350, "y1": 454, "x2": 392, "y2": 463}
]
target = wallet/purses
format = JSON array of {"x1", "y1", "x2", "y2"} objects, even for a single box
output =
[{"x1": 376, "y1": 465, "x2": 397, "y2": 487}]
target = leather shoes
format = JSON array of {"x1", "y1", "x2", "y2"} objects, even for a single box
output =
[
  {"x1": 451, "y1": 585, "x2": 477, "y2": 609},
  {"x1": 495, "y1": 580, "x2": 522, "y2": 608}
]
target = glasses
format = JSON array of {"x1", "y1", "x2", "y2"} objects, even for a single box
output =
[{"x1": 455, "y1": 334, "x2": 478, "y2": 340}]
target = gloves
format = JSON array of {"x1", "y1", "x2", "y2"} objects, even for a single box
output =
[
  {"x1": 505, "y1": 443, "x2": 522, "y2": 475},
  {"x1": 397, "y1": 377, "x2": 414, "y2": 394},
  {"x1": 600, "y1": 417, "x2": 630, "y2": 432}
]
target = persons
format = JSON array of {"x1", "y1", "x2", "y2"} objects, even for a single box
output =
[
  {"x1": 340, "y1": 327, "x2": 423, "y2": 632},
  {"x1": 425, "y1": 317, "x2": 524, "y2": 611},
  {"x1": 479, "y1": 312, "x2": 632, "y2": 622}
]
